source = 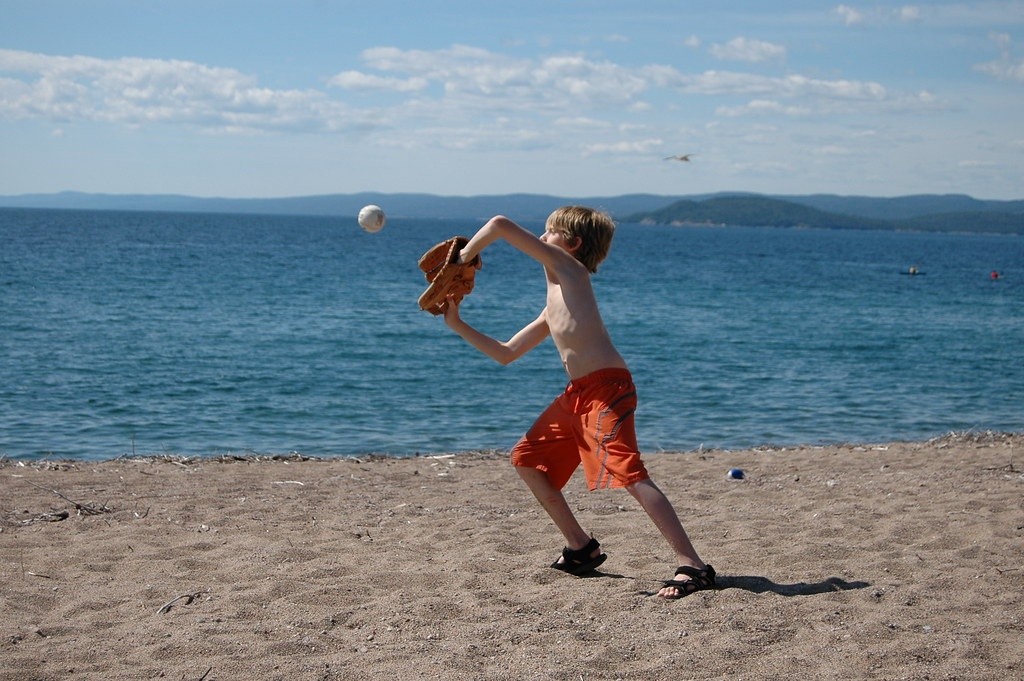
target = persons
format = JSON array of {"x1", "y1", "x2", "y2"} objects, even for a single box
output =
[{"x1": 444, "y1": 206, "x2": 717, "y2": 600}]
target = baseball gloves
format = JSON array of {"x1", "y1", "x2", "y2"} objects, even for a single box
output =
[{"x1": 418, "y1": 238, "x2": 485, "y2": 317}]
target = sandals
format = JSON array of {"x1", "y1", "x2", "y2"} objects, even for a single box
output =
[
  {"x1": 548, "y1": 531, "x2": 607, "y2": 576},
  {"x1": 660, "y1": 564, "x2": 716, "y2": 599}
]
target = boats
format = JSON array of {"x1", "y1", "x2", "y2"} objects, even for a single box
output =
[{"x1": 898, "y1": 270, "x2": 927, "y2": 275}]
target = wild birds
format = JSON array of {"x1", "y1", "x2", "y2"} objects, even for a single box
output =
[{"x1": 664, "y1": 154, "x2": 690, "y2": 161}]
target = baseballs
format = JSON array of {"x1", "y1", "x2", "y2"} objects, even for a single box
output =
[{"x1": 357, "y1": 204, "x2": 385, "y2": 233}]
